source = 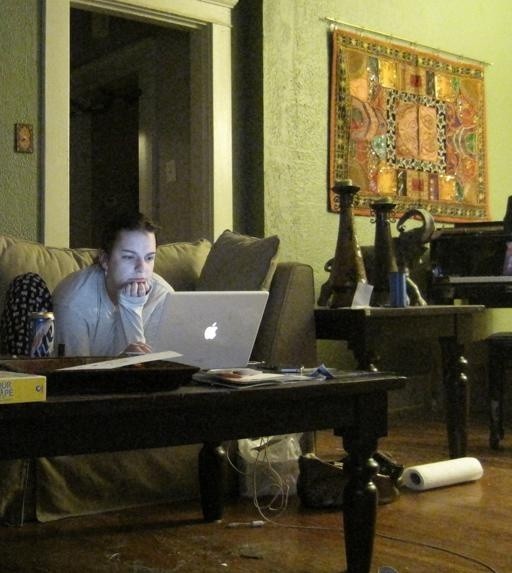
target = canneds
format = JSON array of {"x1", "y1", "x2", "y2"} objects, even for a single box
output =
[{"x1": 26, "y1": 311, "x2": 55, "y2": 360}]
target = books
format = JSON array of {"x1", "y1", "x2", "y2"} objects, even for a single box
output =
[{"x1": 190, "y1": 365, "x2": 317, "y2": 393}]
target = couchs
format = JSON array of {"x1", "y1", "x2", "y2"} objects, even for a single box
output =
[{"x1": 0, "y1": 235, "x2": 317, "y2": 455}]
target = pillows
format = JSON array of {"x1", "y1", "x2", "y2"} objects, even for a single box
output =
[{"x1": 196, "y1": 229, "x2": 281, "y2": 290}]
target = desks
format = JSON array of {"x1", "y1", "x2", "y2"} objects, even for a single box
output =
[{"x1": 316, "y1": 306, "x2": 484, "y2": 458}]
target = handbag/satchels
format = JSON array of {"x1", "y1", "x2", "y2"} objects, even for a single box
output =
[{"x1": 295, "y1": 449, "x2": 404, "y2": 510}]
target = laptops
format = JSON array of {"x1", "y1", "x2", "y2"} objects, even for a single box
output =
[{"x1": 152, "y1": 290, "x2": 269, "y2": 370}]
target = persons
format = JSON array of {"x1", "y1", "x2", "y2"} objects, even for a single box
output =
[{"x1": 48, "y1": 210, "x2": 178, "y2": 358}]
